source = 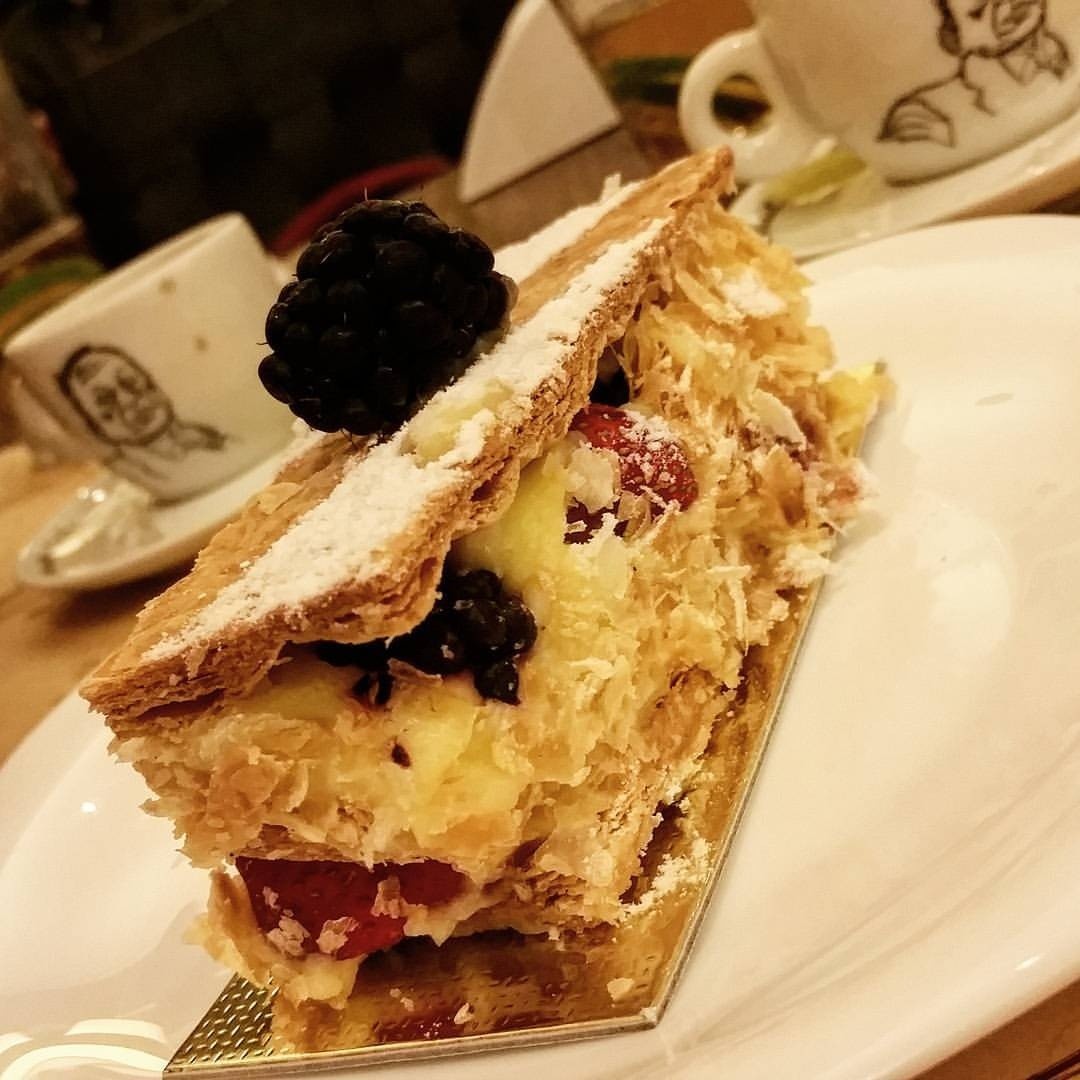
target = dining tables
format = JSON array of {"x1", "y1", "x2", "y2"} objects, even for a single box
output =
[{"x1": 0, "y1": 99, "x2": 1080, "y2": 1080}]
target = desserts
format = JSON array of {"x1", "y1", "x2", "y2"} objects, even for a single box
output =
[{"x1": 77, "y1": 144, "x2": 897, "y2": 1006}]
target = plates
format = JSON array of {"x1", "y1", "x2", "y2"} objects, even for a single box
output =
[
  {"x1": 729, "y1": 106, "x2": 1080, "y2": 260},
  {"x1": 14, "y1": 427, "x2": 328, "y2": 594},
  {"x1": 1, "y1": 209, "x2": 1080, "y2": 1079}
]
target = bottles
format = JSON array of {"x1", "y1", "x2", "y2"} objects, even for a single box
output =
[{"x1": 0, "y1": 52, "x2": 108, "y2": 470}]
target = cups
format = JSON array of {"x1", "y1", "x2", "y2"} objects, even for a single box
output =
[
  {"x1": 676, "y1": 1, "x2": 1080, "y2": 194},
  {"x1": 0, "y1": 209, "x2": 300, "y2": 502}
]
